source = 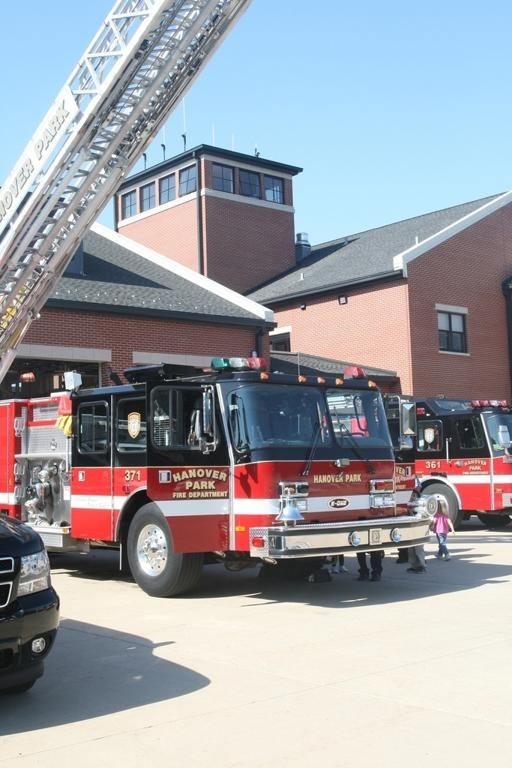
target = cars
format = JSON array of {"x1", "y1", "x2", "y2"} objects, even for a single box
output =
[{"x1": 1, "y1": 509, "x2": 60, "y2": 694}]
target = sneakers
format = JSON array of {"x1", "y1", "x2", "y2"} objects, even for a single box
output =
[{"x1": 328, "y1": 552, "x2": 451, "y2": 584}]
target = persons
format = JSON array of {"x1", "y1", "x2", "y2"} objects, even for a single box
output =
[
  {"x1": 427, "y1": 500, "x2": 456, "y2": 561},
  {"x1": 329, "y1": 543, "x2": 427, "y2": 582}
]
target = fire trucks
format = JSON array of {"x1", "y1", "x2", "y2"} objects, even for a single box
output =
[
  {"x1": 317, "y1": 392, "x2": 511, "y2": 534},
  {"x1": 1, "y1": 0, "x2": 438, "y2": 597}
]
